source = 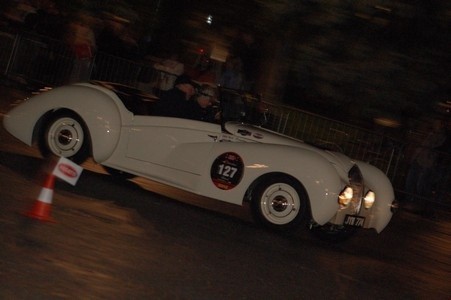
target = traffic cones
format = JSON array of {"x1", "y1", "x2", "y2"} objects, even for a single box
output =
[{"x1": 21, "y1": 170, "x2": 59, "y2": 223}]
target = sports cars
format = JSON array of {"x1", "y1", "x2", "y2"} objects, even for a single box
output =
[{"x1": 2, "y1": 80, "x2": 398, "y2": 235}]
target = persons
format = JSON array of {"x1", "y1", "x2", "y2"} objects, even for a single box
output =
[
  {"x1": 152, "y1": 49, "x2": 184, "y2": 95},
  {"x1": 149, "y1": 75, "x2": 201, "y2": 122},
  {"x1": 14, "y1": 3, "x2": 65, "y2": 95},
  {"x1": 68, "y1": 9, "x2": 103, "y2": 90},
  {"x1": 398, "y1": 115, "x2": 448, "y2": 207},
  {"x1": 184, "y1": 81, "x2": 220, "y2": 125}
]
